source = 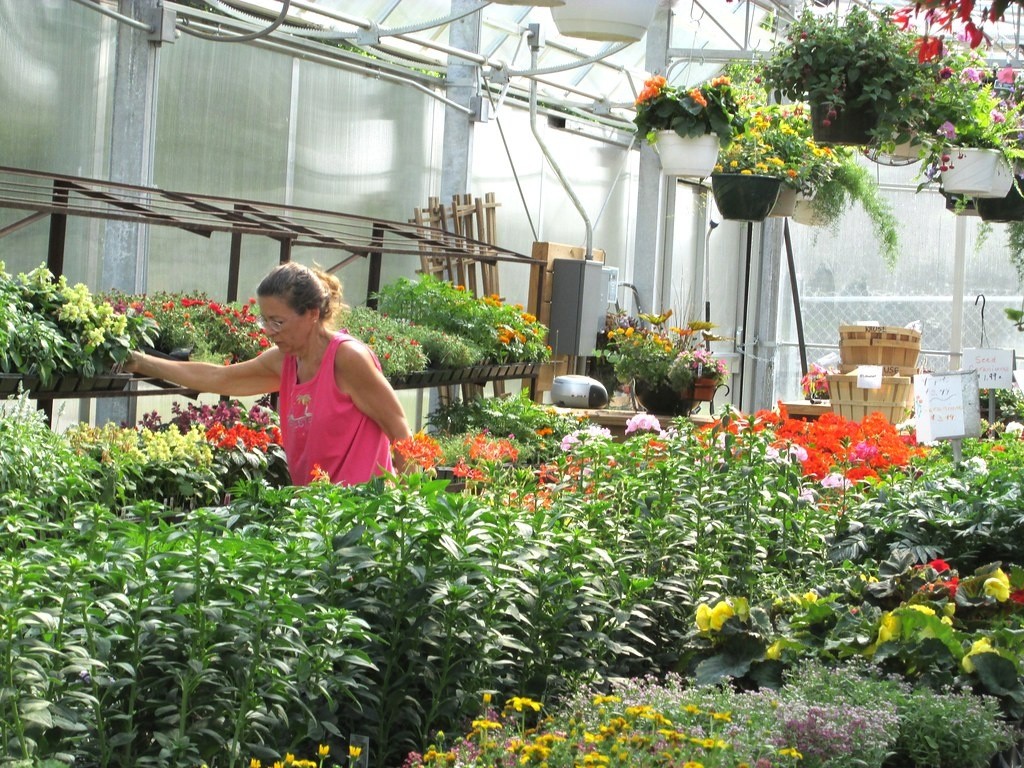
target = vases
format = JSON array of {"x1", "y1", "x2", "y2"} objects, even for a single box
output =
[
  {"x1": 769, "y1": 182, "x2": 797, "y2": 217},
  {"x1": 0, "y1": 362, "x2": 541, "y2": 392},
  {"x1": 635, "y1": 377, "x2": 681, "y2": 416},
  {"x1": 945, "y1": 196, "x2": 980, "y2": 217},
  {"x1": 883, "y1": 125, "x2": 924, "y2": 159},
  {"x1": 940, "y1": 147, "x2": 1018, "y2": 199},
  {"x1": 487, "y1": 1, "x2": 664, "y2": 44},
  {"x1": 711, "y1": 172, "x2": 784, "y2": 223},
  {"x1": 974, "y1": 174, "x2": 1024, "y2": 222},
  {"x1": 653, "y1": 130, "x2": 720, "y2": 177},
  {"x1": 686, "y1": 380, "x2": 718, "y2": 401},
  {"x1": 792, "y1": 198, "x2": 830, "y2": 226},
  {"x1": 808, "y1": 90, "x2": 880, "y2": 146}
]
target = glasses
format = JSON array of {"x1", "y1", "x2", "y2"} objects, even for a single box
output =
[{"x1": 257, "y1": 310, "x2": 300, "y2": 333}]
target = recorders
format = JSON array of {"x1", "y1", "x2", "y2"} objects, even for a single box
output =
[{"x1": 551, "y1": 330, "x2": 608, "y2": 408}]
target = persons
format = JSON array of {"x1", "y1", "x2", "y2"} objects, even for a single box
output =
[{"x1": 119, "y1": 261, "x2": 418, "y2": 488}]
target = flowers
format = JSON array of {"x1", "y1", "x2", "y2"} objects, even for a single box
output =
[
  {"x1": 634, "y1": 0, "x2": 1024, "y2": 271},
  {"x1": 593, "y1": 309, "x2": 738, "y2": 393},
  {"x1": 0, "y1": 260, "x2": 553, "y2": 376}
]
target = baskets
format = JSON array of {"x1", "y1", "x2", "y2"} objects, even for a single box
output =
[{"x1": 826, "y1": 324, "x2": 924, "y2": 427}]
